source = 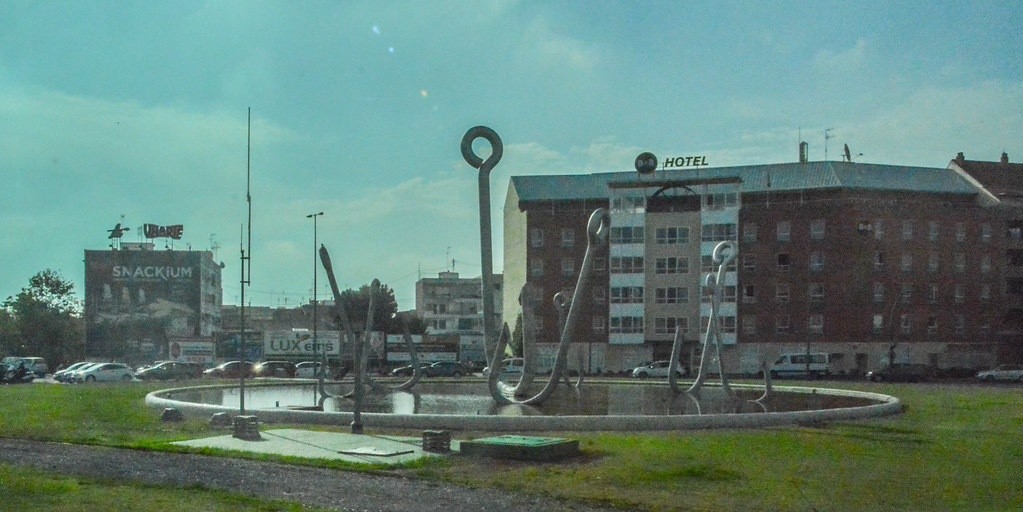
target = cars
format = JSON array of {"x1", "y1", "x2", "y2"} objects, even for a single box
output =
[
  {"x1": 134, "y1": 360, "x2": 203, "y2": 381},
  {"x1": 412, "y1": 361, "x2": 467, "y2": 378},
  {"x1": 64, "y1": 363, "x2": 97, "y2": 383},
  {"x1": 630, "y1": 359, "x2": 688, "y2": 380},
  {"x1": 391, "y1": 361, "x2": 433, "y2": 377},
  {"x1": 864, "y1": 364, "x2": 921, "y2": 384},
  {"x1": 71, "y1": 363, "x2": 135, "y2": 383},
  {"x1": 251, "y1": 360, "x2": 296, "y2": 378},
  {"x1": 53, "y1": 361, "x2": 87, "y2": 382},
  {"x1": 293, "y1": 361, "x2": 331, "y2": 379},
  {"x1": 973, "y1": 363, "x2": 1023, "y2": 385},
  {"x1": 482, "y1": 356, "x2": 526, "y2": 375},
  {"x1": 205, "y1": 362, "x2": 255, "y2": 378}
]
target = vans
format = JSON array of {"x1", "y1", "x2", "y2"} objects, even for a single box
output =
[{"x1": 768, "y1": 352, "x2": 833, "y2": 379}]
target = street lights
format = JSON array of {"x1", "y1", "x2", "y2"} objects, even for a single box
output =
[{"x1": 307, "y1": 211, "x2": 326, "y2": 374}]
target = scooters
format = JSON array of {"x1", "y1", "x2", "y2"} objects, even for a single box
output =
[{"x1": 4, "y1": 363, "x2": 36, "y2": 384}]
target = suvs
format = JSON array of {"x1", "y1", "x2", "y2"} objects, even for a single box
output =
[{"x1": 0, "y1": 356, "x2": 49, "y2": 378}]
